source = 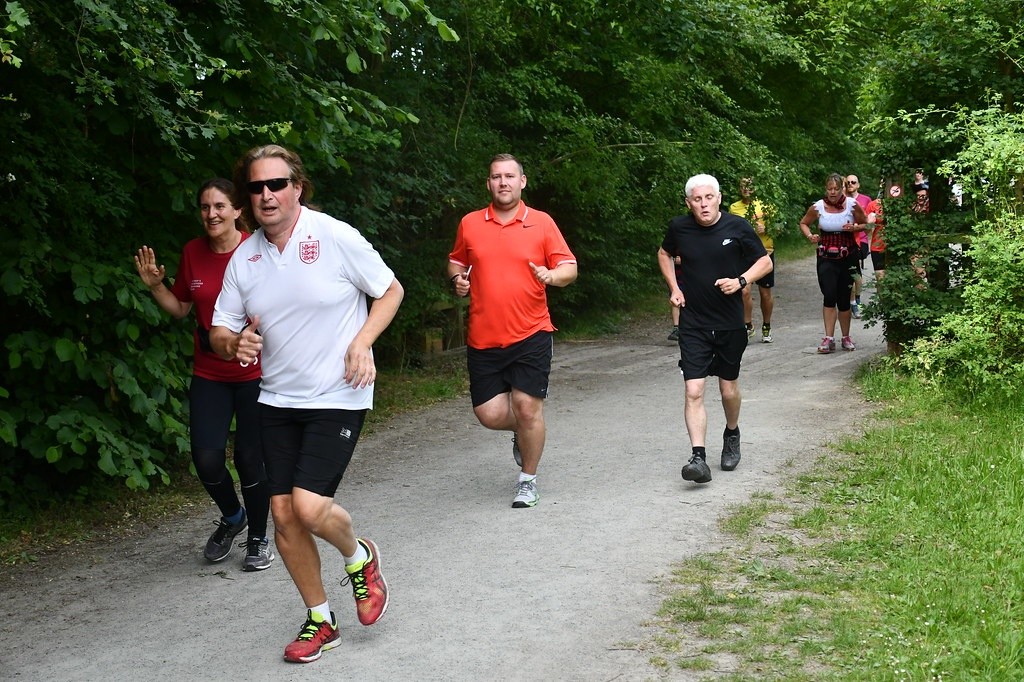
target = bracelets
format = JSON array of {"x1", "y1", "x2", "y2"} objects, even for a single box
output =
[
  {"x1": 806, "y1": 234, "x2": 812, "y2": 238},
  {"x1": 450, "y1": 274, "x2": 459, "y2": 282}
]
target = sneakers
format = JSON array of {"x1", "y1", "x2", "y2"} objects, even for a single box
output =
[
  {"x1": 682, "y1": 452, "x2": 711, "y2": 483},
  {"x1": 512, "y1": 476, "x2": 540, "y2": 508},
  {"x1": 511, "y1": 431, "x2": 523, "y2": 466},
  {"x1": 851, "y1": 304, "x2": 860, "y2": 318},
  {"x1": 744, "y1": 324, "x2": 755, "y2": 338},
  {"x1": 818, "y1": 338, "x2": 835, "y2": 353},
  {"x1": 761, "y1": 326, "x2": 773, "y2": 343},
  {"x1": 721, "y1": 428, "x2": 741, "y2": 471},
  {"x1": 204, "y1": 507, "x2": 250, "y2": 562},
  {"x1": 841, "y1": 337, "x2": 856, "y2": 350},
  {"x1": 237, "y1": 537, "x2": 275, "y2": 571},
  {"x1": 285, "y1": 609, "x2": 342, "y2": 663},
  {"x1": 340, "y1": 538, "x2": 390, "y2": 626},
  {"x1": 668, "y1": 327, "x2": 679, "y2": 340}
]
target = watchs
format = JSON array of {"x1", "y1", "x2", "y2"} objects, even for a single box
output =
[{"x1": 738, "y1": 276, "x2": 747, "y2": 289}]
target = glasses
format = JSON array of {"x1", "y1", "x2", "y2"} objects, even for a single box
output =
[
  {"x1": 848, "y1": 181, "x2": 856, "y2": 185},
  {"x1": 247, "y1": 178, "x2": 296, "y2": 194}
]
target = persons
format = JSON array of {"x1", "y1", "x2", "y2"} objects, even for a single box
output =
[
  {"x1": 667, "y1": 256, "x2": 681, "y2": 341},
  {"x1": 209, "y1": 143, "x2": 405, "y2": 664},
  {"x1": 839, "y1": 174, "x2": 888, "y2": 319},
  {"x1": 447, "y1": 154, "x2": 579, "y2": 507},
  {"x1": 799, "y1": 173, "x2": 868, "y2": 352},
  {"x1": 910, "y1": 166, "x2": 932, "y2": 290},
  {"x1": 658, "y1": 173, "x2": 773, "y2": 485},
  {"x1": 135, "y1": 181, "x2": 275, "y2": 572},
  {"x1": 729, "y1": 175, "x2": 775, "y2": 343}
]
